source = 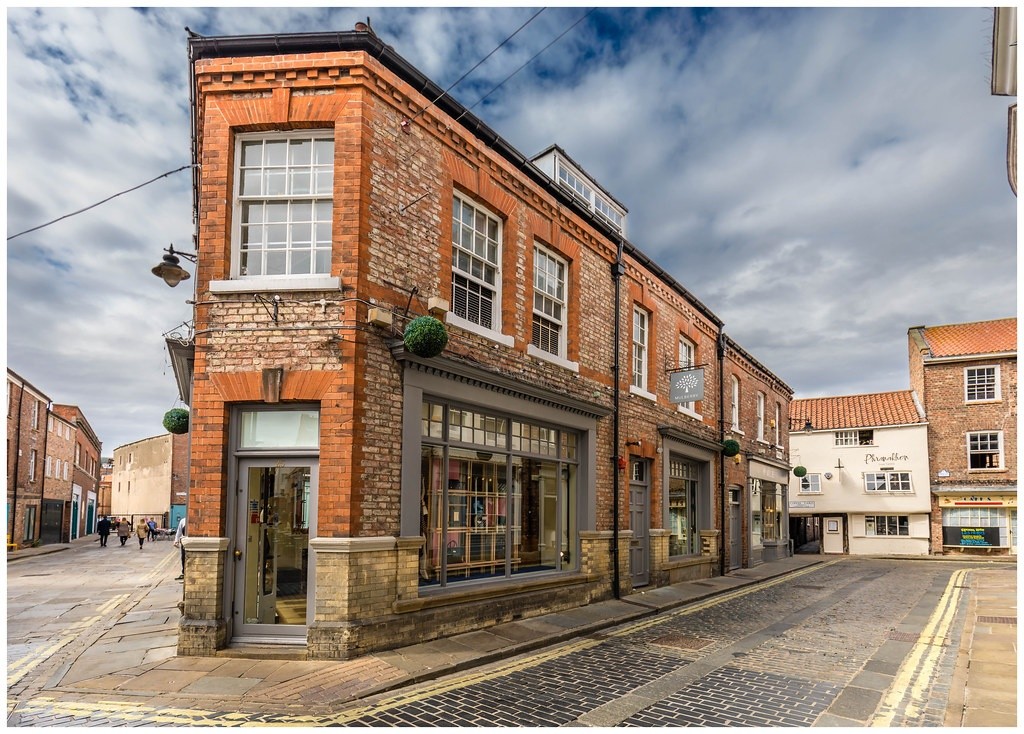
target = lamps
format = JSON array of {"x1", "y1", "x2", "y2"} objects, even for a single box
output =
[
  {"x1": 151, "y1": 243, "x2": 197, "y2": 287},
  {"x1": 626, "y1": 440, "x2": 641, "y2": 446},
  {"x1": 788, "y1": 417, "x2": 813, "y2": 436}
]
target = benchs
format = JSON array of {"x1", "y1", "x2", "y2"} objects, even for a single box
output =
[{"x1": 943, "y1": 545, "x2": 1011, "y2": 553}]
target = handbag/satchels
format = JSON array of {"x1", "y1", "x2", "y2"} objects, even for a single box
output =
[{"x1": 447, "y1": 539, "x2": 463, "y2": 564}]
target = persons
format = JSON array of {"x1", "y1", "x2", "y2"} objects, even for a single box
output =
[
  {"x1": 174, "y1": 518, "x2": 186, "y2": 579},
  {"x1": 147, "y1": 517, "x2": 157, "y2": 541},
  {"x1": 134, "y1": 519, "x2": 147, "y2": 549},
  {"x1": 117, "y1": 518, "x2": 131, "y2": 546},
  {"x1": 98, "y1": 516, "x2": 110, "y2": 547}
]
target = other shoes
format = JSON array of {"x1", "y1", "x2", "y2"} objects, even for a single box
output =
[
  {"x1": 121, "y1": 543, "x2": 125, "y2": 546},
  {"x1": 140, "y1": 545, "x2": 143, "y2": 549},
  {"x1": 175, "y1": 575, "x2": 184, "y2": 580},
  {"x1": 103, "y1": 544, "x2": 106, "y2": 547},
  {"x1": 100, "y1": 544, "x2": 104, "y2": 547}
]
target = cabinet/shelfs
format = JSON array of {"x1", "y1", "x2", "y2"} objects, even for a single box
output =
[{"x1": 436, "y1": 490, "x2": 523, "y2": 581}]
